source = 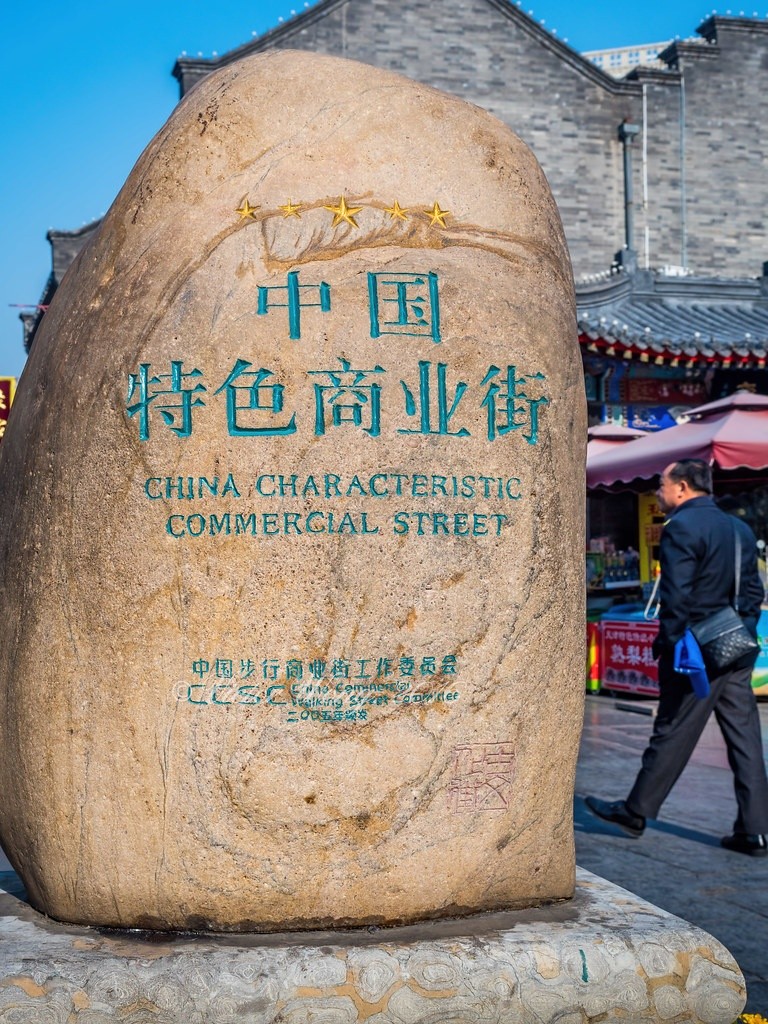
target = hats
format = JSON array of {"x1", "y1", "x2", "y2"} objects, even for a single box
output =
[{"x1": 673, "y1": 625, "x2": 711, "y2": 699}]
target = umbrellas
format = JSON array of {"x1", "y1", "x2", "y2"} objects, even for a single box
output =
[{"x1": 585, "y1": 390, "x2": 768, "y2": 501}]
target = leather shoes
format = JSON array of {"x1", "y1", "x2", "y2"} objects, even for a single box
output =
[
  {"x1": 720, "y1": 834, "x2": 768, "y2": 856},
  {"x1": 584, "y1": 796, "x2": 646, "y2": 836}
]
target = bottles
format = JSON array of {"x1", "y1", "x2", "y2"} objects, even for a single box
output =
[{"x1": 603, "y1": 546, "x2": 639, "y2": 582}]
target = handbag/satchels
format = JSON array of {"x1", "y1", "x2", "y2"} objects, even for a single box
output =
[{"x1": 691, "y1": 605, "x2": 760, "y2": 671}]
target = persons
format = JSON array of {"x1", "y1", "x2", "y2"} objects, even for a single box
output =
[{"x1": 583, "y1": 459, "x2": 768, "y2": 855}]
